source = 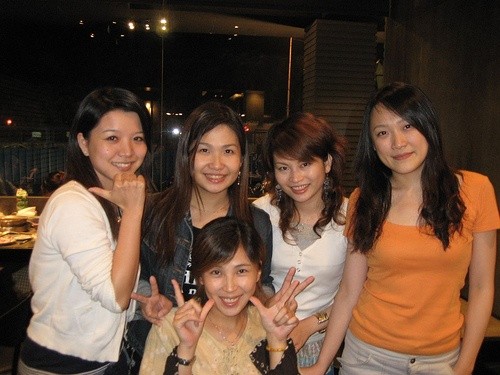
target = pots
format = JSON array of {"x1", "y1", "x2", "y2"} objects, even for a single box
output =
[{"x1": 0, "y1": 215, "x2": 31, "y2": 232}]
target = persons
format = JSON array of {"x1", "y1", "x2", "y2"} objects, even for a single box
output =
[
  {"x1": 0, "y1": 167, "x2": 68, "y2": 195},
  {"x1": 128, "y1": 101, "x2": 315, "y2": 375},
  {"x1": 138, "y1": 216, "x2": 301, "y2": 374},
  {"x1": 16, "y1": 87, "x2": 155, "y2": 375},
  {"x1": 298, "y1": 80, "x2": 499, "y2": 375},
  {"x1": 250, "y1": 111, "x2": 351, "y2": 375}
]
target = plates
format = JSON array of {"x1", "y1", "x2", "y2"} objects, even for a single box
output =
[
  {"x1": 12, "y1": 234, "x2": 32, "y2": 241},
  {"x1": 0, "y1": 237, "x2": 15, "y2": 244}
]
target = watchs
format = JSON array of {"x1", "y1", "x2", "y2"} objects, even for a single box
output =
[{"x1": 171, "y1": 345, "x2": 196, "y2": 366}]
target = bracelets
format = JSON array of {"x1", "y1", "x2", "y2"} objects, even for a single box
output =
[
  {"x1": 312, "y1": 310, "x2": 328, "y2": 334},
  {"x1": 265, "y1": 345, "x2": 288, "y2": 352}
]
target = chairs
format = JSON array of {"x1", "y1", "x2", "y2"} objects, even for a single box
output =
[{"x1": 0, "y1": 290, "x2": 34, "y2": 375}]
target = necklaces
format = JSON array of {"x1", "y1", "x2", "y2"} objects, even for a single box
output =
[
  {"x1": 204, "y1": 310, "x2": 247, "y2": 347},
  {"x1": 190, "y1": 202, "x2": 229, "y2": 219},
  {"x1": 291, "y1": 200, "x2": 324, "y2": 231}
]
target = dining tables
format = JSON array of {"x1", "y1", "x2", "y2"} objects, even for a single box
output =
[{"x1": 0, "y1": 227, "x2": 37, "y2": 249}]
